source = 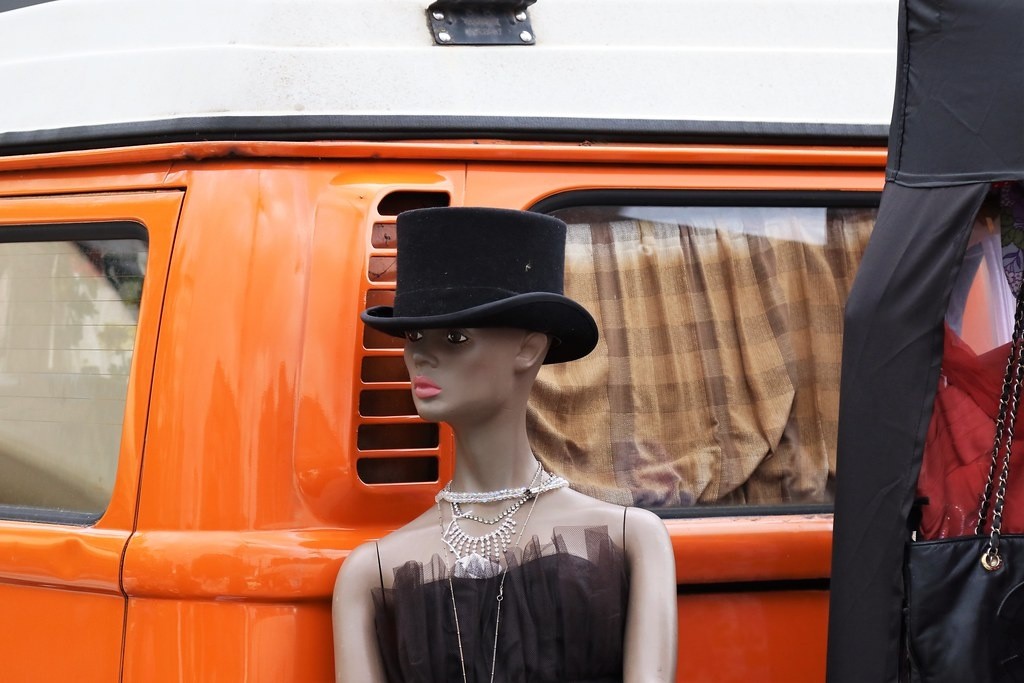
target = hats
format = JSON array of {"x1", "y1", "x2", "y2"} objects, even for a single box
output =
[{"x1": 361, "y1": 205, "x2": 599, "y2": 365}]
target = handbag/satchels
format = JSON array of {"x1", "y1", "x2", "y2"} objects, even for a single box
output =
[{"x1": 904, "y1": 529, "x2": 1024, "y2": 682}]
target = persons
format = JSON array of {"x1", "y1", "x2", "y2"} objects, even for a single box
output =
[{"x1": 332, "y1": 206, "x2": 679, "y2": 683}]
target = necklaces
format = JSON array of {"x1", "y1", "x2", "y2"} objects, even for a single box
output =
[{"x1": 435, "y1": 461, "x2": 570, "y2": 683}]
